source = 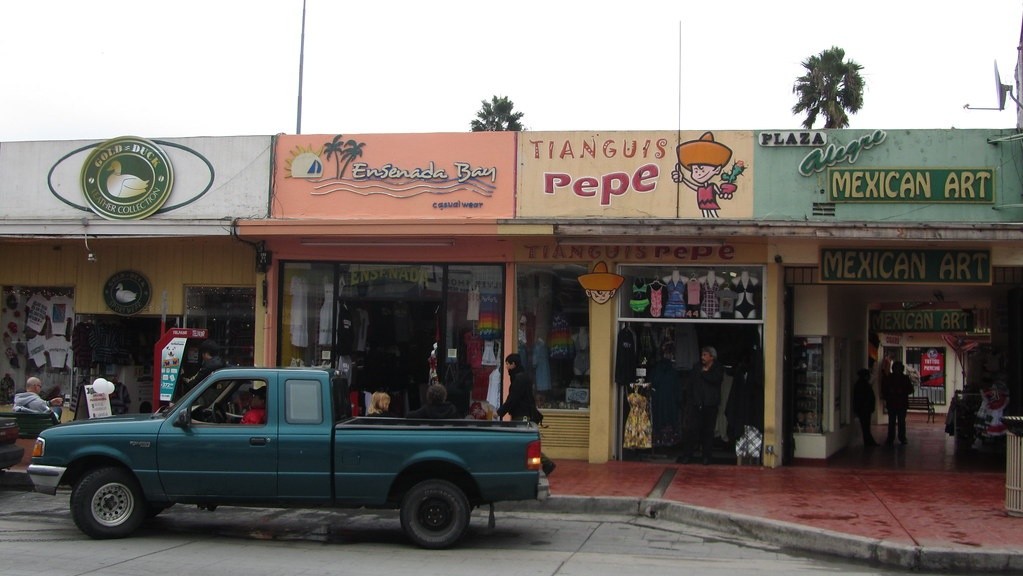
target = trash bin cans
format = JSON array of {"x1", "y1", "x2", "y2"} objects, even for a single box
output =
[{"x1": 999, "y1": 416, "x2": 1023, "y2": 518}]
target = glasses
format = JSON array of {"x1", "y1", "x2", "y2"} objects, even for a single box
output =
[
  {"x1": 37, "y1": 384, "x2": 42, "y2": 389},
  {"x1": 250, "y1": 394, "x2": 255, "y2": 397}
]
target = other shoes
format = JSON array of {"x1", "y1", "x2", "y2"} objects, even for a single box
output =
[{"x1": 544, "y1": 463, "x2": 555, "y2": 476}]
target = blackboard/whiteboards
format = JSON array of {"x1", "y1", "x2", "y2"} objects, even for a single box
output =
[{"x1": 73, "y1": 384, "x2": 111, "y2": 422}]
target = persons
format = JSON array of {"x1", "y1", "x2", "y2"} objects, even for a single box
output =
[
  {"x1": 406, "y1": 384, "x2": 460, "y2": 419},
  {"x1": 853, "y1": 368, "x2": 880, "y2": 446},
  {"x1": 885, "y1": 361, "x2": 913, "y2": 444},
  {"x1": 184, "y1": 339, "x2": 225, "y2": 405},
  {"x1": 14, "y1": 377, "x2": 63, "y2": 420},
  {"x1": 493, "y1": 354, "x2": 556, "y2": 476},
  {"x1": 240, "y1": 386, "x2": 267, "y2": 424},
  {"x1": 682, "y1": 346, "x2": 722, "y2": 465},
  {"x1": 367, "y1": 392, "x2": 395, "y2": 417}
]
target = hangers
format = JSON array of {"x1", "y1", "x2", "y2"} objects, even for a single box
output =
[
  {"x1": 689, "y1": 272, "x2": 697, "y2": 281},
  {"x1": 647, "y1": 274, "x2": 670, "y2": 287}
]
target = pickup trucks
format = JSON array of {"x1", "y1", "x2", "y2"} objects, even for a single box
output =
[{"x1": 27, "y1": 367, "x2": 551, "y2": 550}]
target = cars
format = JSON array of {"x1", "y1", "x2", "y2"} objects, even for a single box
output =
[{"x1": 0, "y1": 417, "x2": 25, "y2": 469}]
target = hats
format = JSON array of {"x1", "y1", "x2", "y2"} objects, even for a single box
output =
[
  {"x1": 702, "y1": 347, "x2": 718, "y2": 361},
  {"x1": 249, "y1": 386, "x2": 266, "y2": 399}
]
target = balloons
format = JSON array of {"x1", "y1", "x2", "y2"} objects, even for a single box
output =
[{"x1": 93, "y1": 378, "x2": 115, "y2": 394}]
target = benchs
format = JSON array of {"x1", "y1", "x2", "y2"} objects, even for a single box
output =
[
  {"x1": 906, "y1": 396, "x2": 936, "y2": 423},
  {"x1": 0, "y1": 411, "x2": 61, "y2": 439}
]
